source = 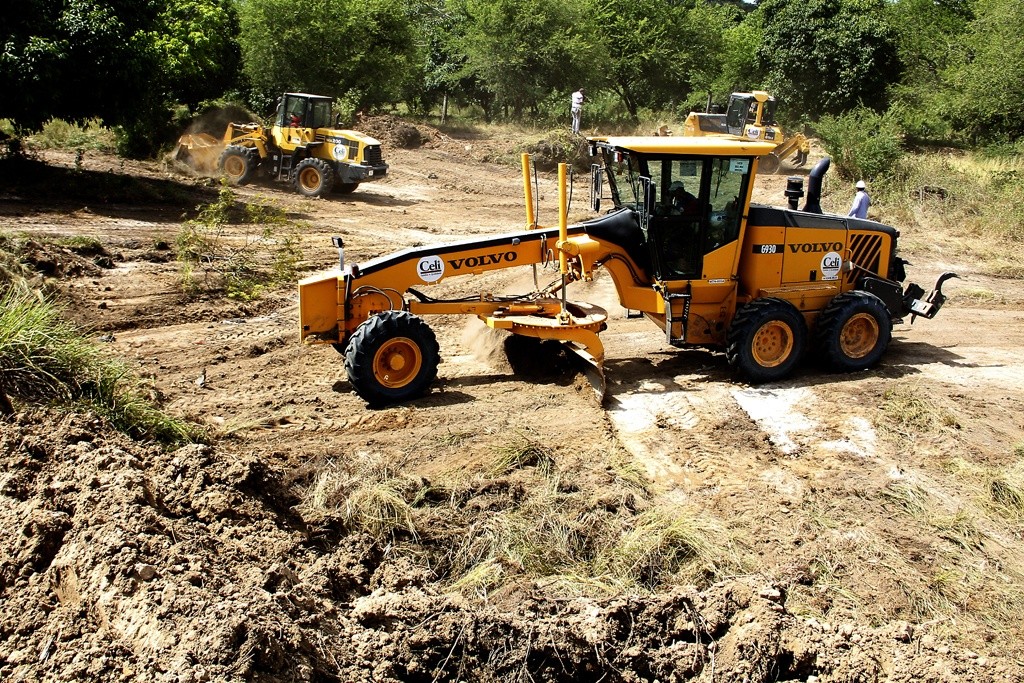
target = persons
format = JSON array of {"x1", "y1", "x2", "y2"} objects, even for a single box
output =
[
  {"x1": 847, "y1": 180, "x2": 872, "y2": 218},
  {"x1": 571, "y1": 88, "x2": 585, "y2": 135},
  {"x1": 658, "y1": 181, "x2": 699, "y2": 244}
]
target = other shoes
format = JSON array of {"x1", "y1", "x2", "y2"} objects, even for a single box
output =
[{"x1": 572, "y1": 131, "x2": 579, "y2": 134}]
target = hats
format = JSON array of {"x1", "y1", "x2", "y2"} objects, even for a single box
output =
[
  {"x1": 668, "y1": 181, "x2": 684, "y2": 190},
  {"x1": 856, "y1": 181, "x2": 865, "y2": 189}
]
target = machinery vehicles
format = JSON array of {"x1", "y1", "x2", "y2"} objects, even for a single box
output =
[
  {"x1": 296, "y1": 91, "x2": 962, "y2": 411},
  {"x1": 681, "y1": 88, "x2": 810, "y2": 177},
  {"x1": 176, "y1": 91, "x2": 391, "y2": 197}
]
